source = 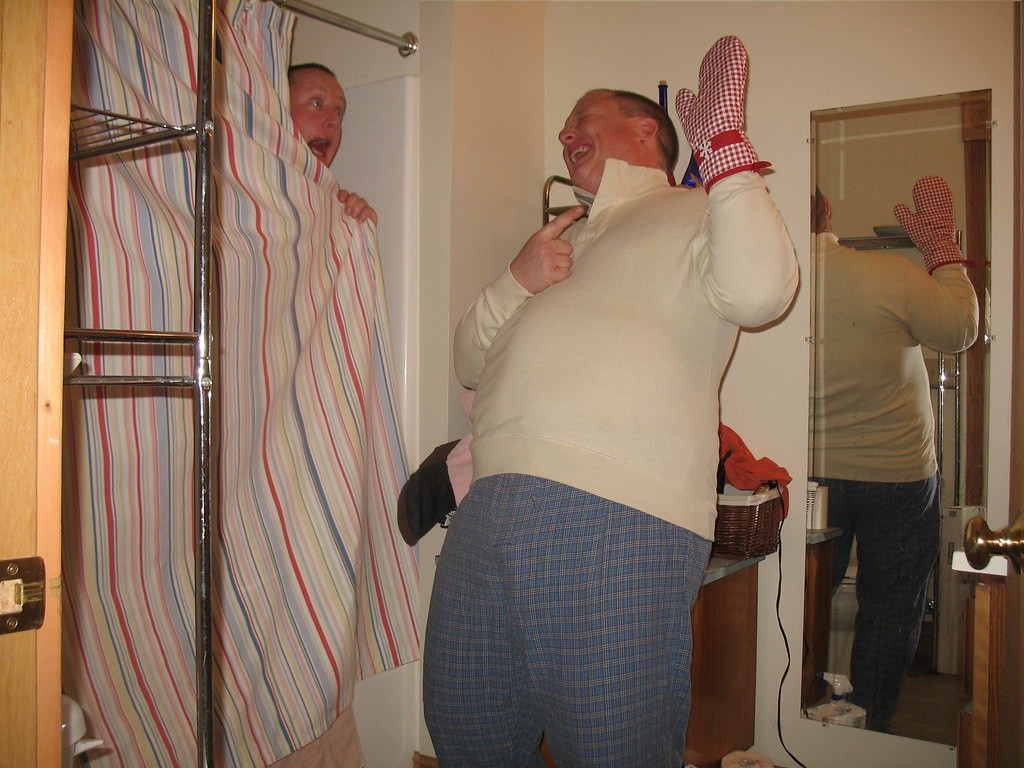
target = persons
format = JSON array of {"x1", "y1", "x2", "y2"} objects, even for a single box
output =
[
  {"x1": 269, "y1": 63, "x2": 379, "y2": 768},
  {"x1": 809, "y1": 178, "x2": 979, "y2": 734},
  {"x1": 423, "y1": 35, "x2": 797, "y2": 768}
]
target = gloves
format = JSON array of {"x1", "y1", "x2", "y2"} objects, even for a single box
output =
[
  {"x1": 676, "y1": 36, "x2": 773, "y2": 195},
  {"x1": 894, "y1": 175, "x2": 979, "y2": 277}
]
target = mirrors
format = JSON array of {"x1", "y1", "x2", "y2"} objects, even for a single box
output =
[{"x1": 799, "y1": 88, "x2": 993, "y2": 749}]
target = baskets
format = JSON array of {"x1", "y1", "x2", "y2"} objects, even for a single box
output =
[{"x1": 711, "y1": 485, "x2": 784, "y2": 560}]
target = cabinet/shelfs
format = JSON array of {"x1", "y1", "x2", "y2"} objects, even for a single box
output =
[
  {"x1": 538, "y1": 556, "x2": 766, "y2": 768},
  {"x1": 802, "y1": 527, "x2": 844, "y2": 708},
  {"x1": 65, "y1": 0, "x2": 218, "y2": 768}
]
